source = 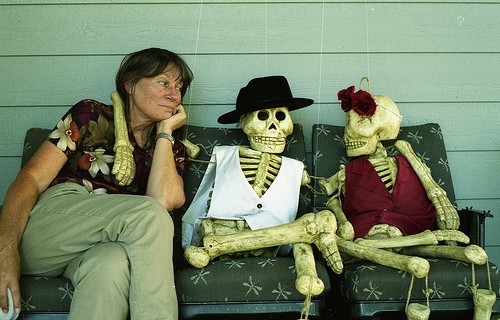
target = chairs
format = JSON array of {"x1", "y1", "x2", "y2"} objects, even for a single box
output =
[{"x1": 20, "y1": 122, "x2": 500, "y2": 320}]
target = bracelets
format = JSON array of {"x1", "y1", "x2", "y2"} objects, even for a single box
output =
[{"x1": 154, "y1": 133, "x2": 175, "y2": 146}]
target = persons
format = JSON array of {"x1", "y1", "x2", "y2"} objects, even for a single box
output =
[
  {"x1": 317, "y1": 78, "x2": 496, "y2": 320},
  {"x1": 0, "y1": 47, "x2": 197, "y2": 320},
  {"x1": 109, "y1": 76, "x2": 345, "y2": 297}
]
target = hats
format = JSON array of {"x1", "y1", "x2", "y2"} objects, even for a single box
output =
[{"x1": 217, "y1": 76, "x2": 315, "y2": 124}]
